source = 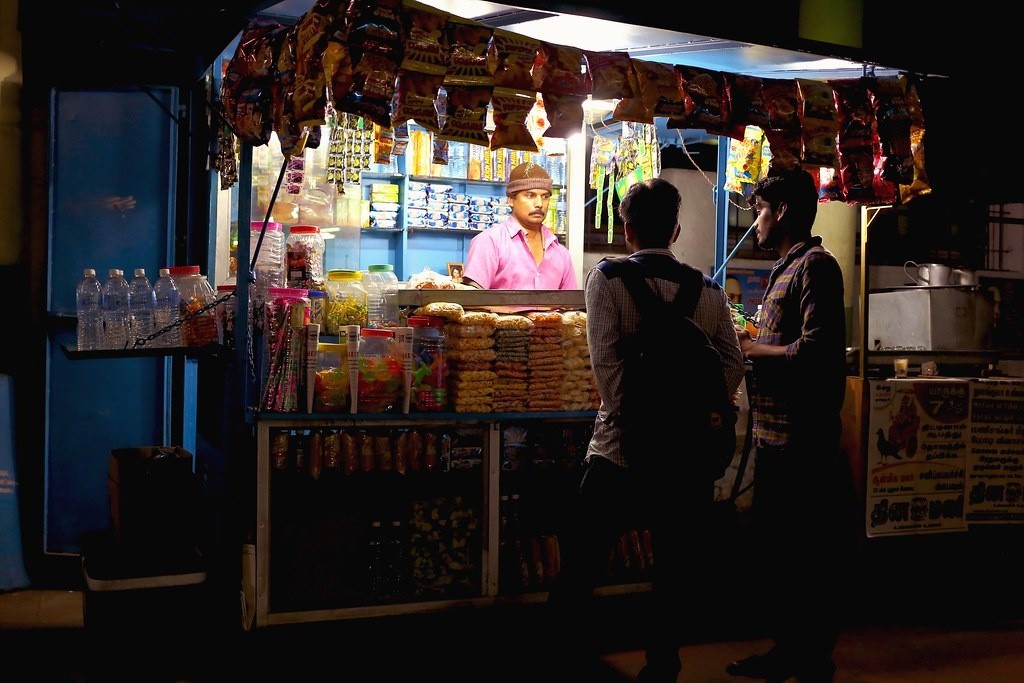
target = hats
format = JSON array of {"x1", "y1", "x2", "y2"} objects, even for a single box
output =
[{"x1": 506, "y1": 162, "x2": 553, "y2": 195}]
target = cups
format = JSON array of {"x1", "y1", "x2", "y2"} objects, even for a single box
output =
[
  {"x1": 928, "y1": 266, "x2": 954, "y2": 285},
  {"x1": 894, "y1": 358, "x2": 908, "y2": 379},
  {"x1": 904, "y1": 261, "x2": 945, "y2": 286}
]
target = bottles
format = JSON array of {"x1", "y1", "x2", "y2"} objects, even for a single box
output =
[
  {"x1": 103, "y1": 269, "x2": 127, "y2": 349},
  {"x1": 118, "y1": 270, "x2": 131, "y2": 341},
  {"x1": 153, "y1": 269, "x2": 181, "y2": 347},
  {"x1": 75, "y1": 268, "x2": 104, "y2": 351},
  {"x1": 754, "y1": 305, "x2": 763, "y2": 326},
  {"x1": 130, "y1": 269, "x2": 157, "y2": 348}
]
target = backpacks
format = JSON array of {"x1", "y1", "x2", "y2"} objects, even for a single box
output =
[{"x1": 605, "y1": 256, "x2": 740, "y2": 481}]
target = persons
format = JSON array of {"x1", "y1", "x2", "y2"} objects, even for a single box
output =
[
  {"x1": 733, "y1": 170, "x2": 848, "y2": 683},
  {"x1": 554, "y1": 177, "x2": 746, "y2": 683},
  {"x1": 464, "y1": 162, "x2": 579, "y2": 290}
]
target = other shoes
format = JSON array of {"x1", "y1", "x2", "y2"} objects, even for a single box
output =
[
  {"x1": 635, "y1": 664, "x2": 682, "y2": 683},
  {"x1": 725, "y1": 644, "x2": 836, "y2": 682}
]
